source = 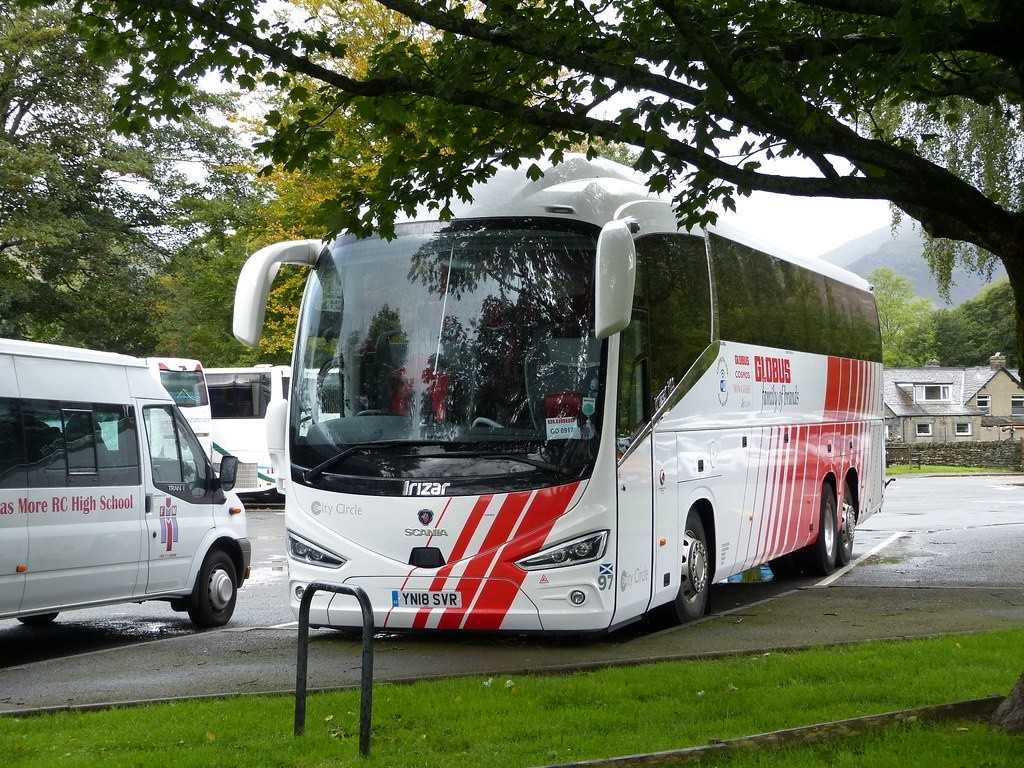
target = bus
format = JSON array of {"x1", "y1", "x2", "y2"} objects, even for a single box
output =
[
  {"x1": 233, "y1": 153, "x2": 885, "y2": 636},
  {"x1": 0, "y1": 338, "x2": 340, "y2": 628}
]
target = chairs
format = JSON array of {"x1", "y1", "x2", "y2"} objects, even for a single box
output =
[
  {"x1": 389, "y1": 352, "x2": 453, "y2": 424},
  {"x1": 532, "y1": 392, "x2": 586, "y2": 430},
  {"x1": 66, "y1": 414, "x2": 113, "y2": 468}
]
